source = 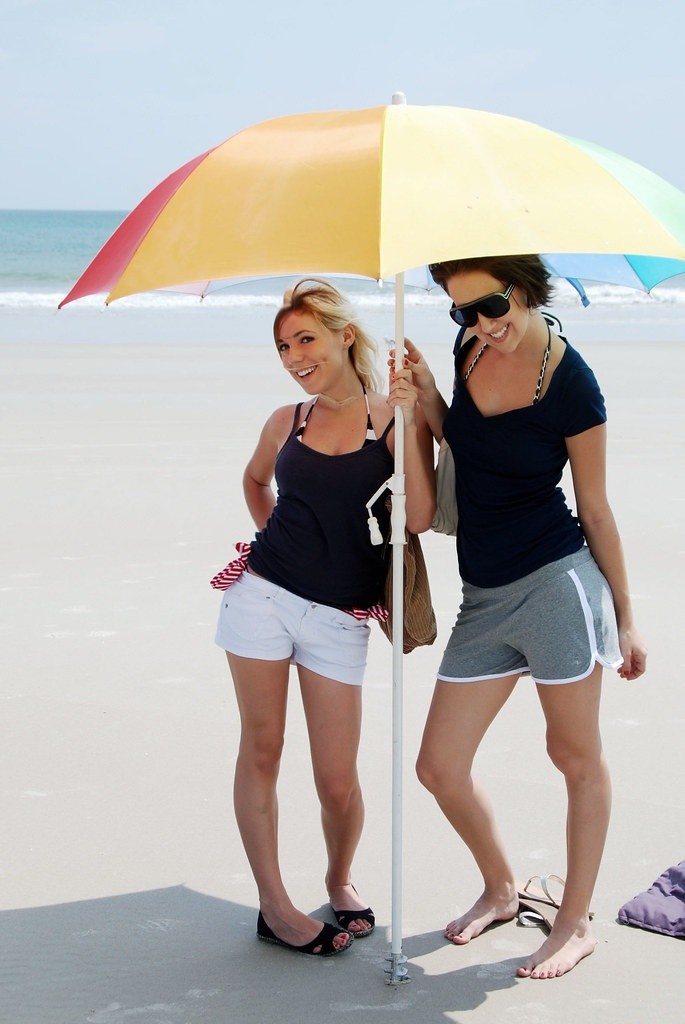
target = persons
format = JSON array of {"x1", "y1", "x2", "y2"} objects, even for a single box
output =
[
  {"x1": 387, "y1": 254, "x2": 647, "y2": 980},
  {"x1": 214, "y1": 277, "x2": 437, "y2": 958}
]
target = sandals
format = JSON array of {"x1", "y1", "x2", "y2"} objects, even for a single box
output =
[
  {"x1": 257, "y1": 910, "x2": 355, "y2": 956},
  {"x1": 330, "y1": 883, "x2": 376, "y2": 938}
]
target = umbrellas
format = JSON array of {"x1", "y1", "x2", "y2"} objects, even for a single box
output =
[{"x1": 56, "y1": 93, "x2": 685, "y2": 982}]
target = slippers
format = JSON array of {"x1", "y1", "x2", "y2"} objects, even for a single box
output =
[
  {"x1": 516, "y1": 874, "x2": 595, "y2": 917},
  {"x1": 519, "y1": 898, "x2": 559, "y2": 930}
]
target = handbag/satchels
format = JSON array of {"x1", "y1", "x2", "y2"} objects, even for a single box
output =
[{"x1": 429, "y1": 439, "x2": 459, "y2": 537}]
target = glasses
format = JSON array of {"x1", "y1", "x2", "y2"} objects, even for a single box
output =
[{"x1": 449, "y1": 283, "x2": 516, "y2": 327}]
